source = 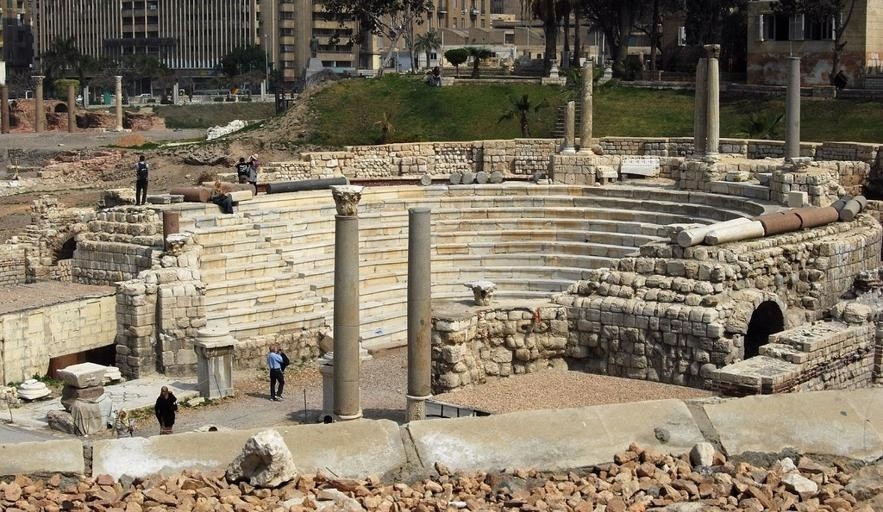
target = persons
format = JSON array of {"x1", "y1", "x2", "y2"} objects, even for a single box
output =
[
  {"x1": 134, "y1": 156, "x2": 149, "y2": 206},
  {"x1": 429, "y1": 66, "x2": 442, "y2": 87},
  {"x1": 155, "y1": 386, "x2": 178, "y2": 435},
  {"x1": 323, "y1": 415, "x2": 333, "y2": 424},
  {"x1": 116, "y1": 411, "x2": 131, "y2": 439},
  {"x1": 233, "y1": 157, "x2": 252, "y2": 184},
  {"x1": 834, "y1": 69, "x2": 848, "y2": 90},
  {"x1": 267, "y1": 346, "x2": 290, "y2": 401},
  {"x1": 246, "y1": 154, "x2": 261, "y2": 196},
  {"x1": 209, "y1": 427, "x2": 217, "y2": 431}
]
